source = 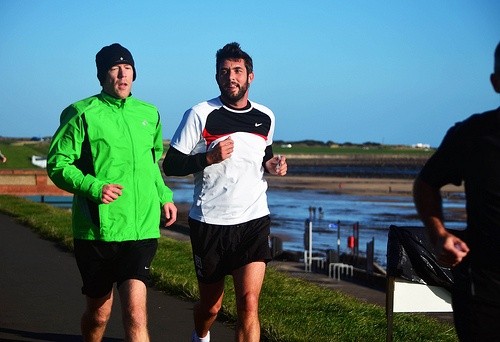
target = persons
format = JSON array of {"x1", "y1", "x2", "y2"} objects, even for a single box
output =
[
  {"x1": 162, "y1": 41, "x2": 287, "y2": 342},
  {"x1": 47, "y1": 43, "x2": 177, "y2": 342},
  {"x1": 413, "y1": 40, "x2": 500, "y2": 342}
]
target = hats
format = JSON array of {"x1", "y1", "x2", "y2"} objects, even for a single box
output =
[{"x1": 95, "y1": 43, "x2": 136, "y2": 84}]
